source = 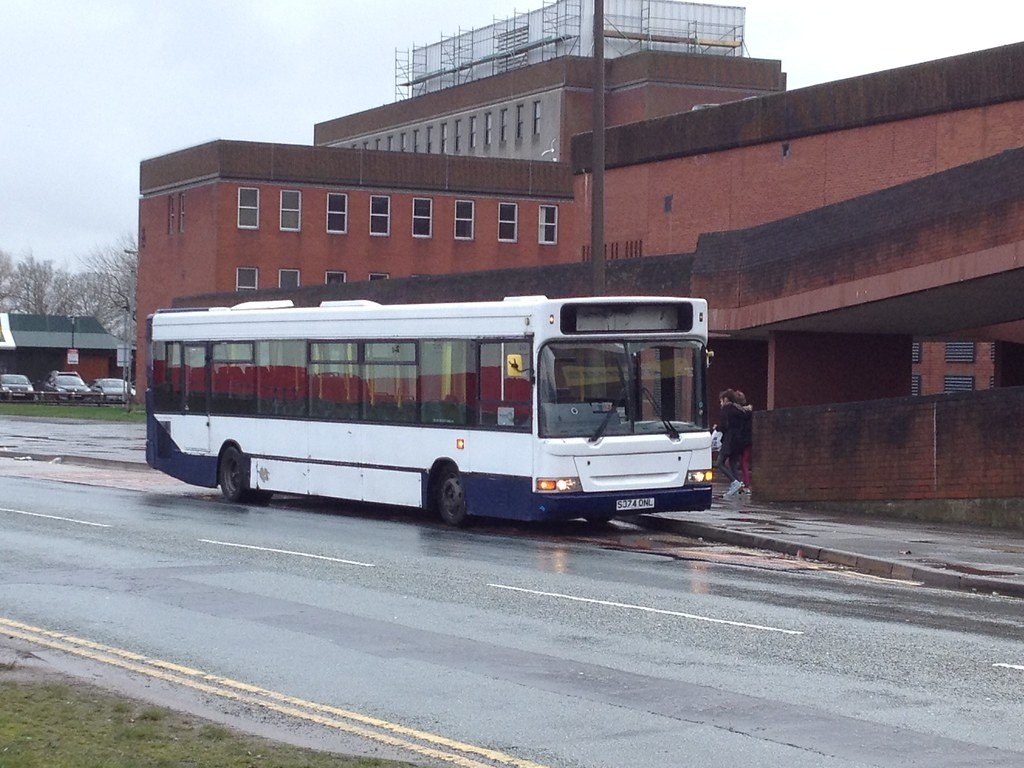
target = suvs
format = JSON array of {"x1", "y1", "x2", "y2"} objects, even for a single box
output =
[{"x1": 41, "y1": 370, "x2": 86, "y2": 390}]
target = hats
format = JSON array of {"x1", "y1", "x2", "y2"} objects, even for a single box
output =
[{"x1": 735, "y1": 391, "x2": 746, "y2": 404}]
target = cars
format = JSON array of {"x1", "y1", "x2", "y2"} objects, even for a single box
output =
[
  {"x1": 0, "y1": 374, "x2": 35, "y2": 401},
  {"x1": 89, "y1": 378, "x2": 136, "y2": 403},
  {"x1": 43, "y1": 376, "x2": 91, "y2": 401}
]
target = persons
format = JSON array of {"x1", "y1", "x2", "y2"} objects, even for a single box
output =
[{"x1": 713, "y1": 389, "x2": 752, "y2": 497}]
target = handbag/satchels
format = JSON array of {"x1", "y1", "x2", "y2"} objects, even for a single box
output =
[{"x1": 712, "y1": 429, "x2": 723, "y2": 451}]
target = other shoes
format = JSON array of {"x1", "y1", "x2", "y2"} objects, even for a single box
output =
[
  {"x1": 735, "y1": 482, "x2": 744, "y2": 494},
  {"x1": 727, "y1": 480, "x2": 741, "y2": 497},
  {"x1": 745, "y1": 489, "x2": 751, "y2": 495}
]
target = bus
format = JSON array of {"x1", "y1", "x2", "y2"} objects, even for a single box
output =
[{"x1": 145, "y1": 297, "x2": 718, "y2": 531}]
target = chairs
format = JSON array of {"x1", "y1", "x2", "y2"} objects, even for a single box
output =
[{"x1": 179, "y1": 389, "x2": 527, "y2": 429}]
